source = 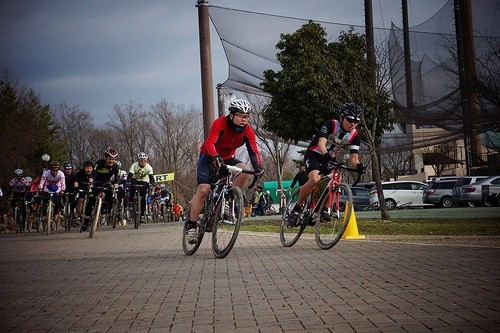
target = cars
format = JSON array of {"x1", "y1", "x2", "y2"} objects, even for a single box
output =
[
  {"x1": 422, "y1": 181, "x2": 453, "y2": 207},
  {"x1": 453, "y1": 176, "x2": 489, "y2": 201},
  {"x1": 338, "y1": 186, "x2": 370, "y2": 212},
  {"x1": 456, "y1": 176, "x2": 500, "y2": 206},
  {"x1": 369, "y1": 180, "x2": 435, "y2": 210}
]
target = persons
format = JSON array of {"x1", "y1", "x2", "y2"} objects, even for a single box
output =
[
  {"x1": 288, "y1": 166, "x2": 308, "y2": 190},
  {"x1": 252, "y1": 188, "x2": 273, "y2": 215},
  {"x1": 186, "y1": 99, "x2": 262, "y2": 243},
  {"x1": 0, "y1": 146, "x2": 186, "y2": 234},
  {"x1": 287, "y1": 103, "x2": 363, "y2": 228}
]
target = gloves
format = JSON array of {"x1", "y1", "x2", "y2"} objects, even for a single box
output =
[
  {"x1": 254, "y1": 166, "x2": 264, "y2": 178},
  {"x1": 323, "y1": 152, "x2": 337, "y2": 168},
  {"x1": 357, "y1": 164, "x2": 366, "y2": 175},
  {"x1": 212, "y1": 157, "x2": 227, "y2": 174}
]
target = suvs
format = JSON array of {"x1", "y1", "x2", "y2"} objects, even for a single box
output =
[{"x1": 356, "y1": 183, "x2": 376, "y2": 190}]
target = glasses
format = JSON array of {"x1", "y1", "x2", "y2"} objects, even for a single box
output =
[
  {"x1": 232, "y1": 112, "x2": 250, "y2": 119},
  {"x1": 343, "y1": 117, "x2": 359, "y2": 124}
]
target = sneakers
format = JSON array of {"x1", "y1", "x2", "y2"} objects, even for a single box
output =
[
  {"x1": 224, "y1": 201, "x2": 232, "y2": 217},
  {"x1": 320, "y1": 212, "x2": 334, "y2": 223},
  {"x1": 186, "y1": 227, "x2": 198, "y2": 244},
  {"x1": 288, "y1": 210, "x2": 300, "y2": 223}
]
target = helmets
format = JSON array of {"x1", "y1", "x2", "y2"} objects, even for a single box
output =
[
  {"x1": 15, "y1": 168, "x2": 23, "y2": 176},
  {"x1": 49, "y1": 161, "x2": 60, "y2": 168},
  {"x1": 229, "y1": 99, "x2": 251, "y2": 114},
  {"x1": 63, "y1": 162, "x2": 72, "y2": 170},
  {"x1": 340, "y1": 102, "x2": 362, "y2": 120},
  {"x1": 104, "y1": 148, "x2": 118, "y2": 158},
  {"x1": 160, "y1": 184, "x2": 165, "y2": 189},
  {"x1": 137, "y1": 152, "x2": 149, "y2": 160}
]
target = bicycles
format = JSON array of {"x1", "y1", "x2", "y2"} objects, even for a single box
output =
[
  {"x1": 181, "y1": 157, "x2": 264, "y2": 258},
  {"x1": 252, "y1": 204, "x2": 276, "y2": 216},
  {"x1": 279, "y1": 162, "x2": 368, "y2": 249},
  {"x1": 0, "y1": 185, "x2": 184, "y2": 238}
]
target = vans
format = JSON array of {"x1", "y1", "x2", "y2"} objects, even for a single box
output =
[{"x1": 431, "y1": 177, "x2": 460, "y2": 181}]
